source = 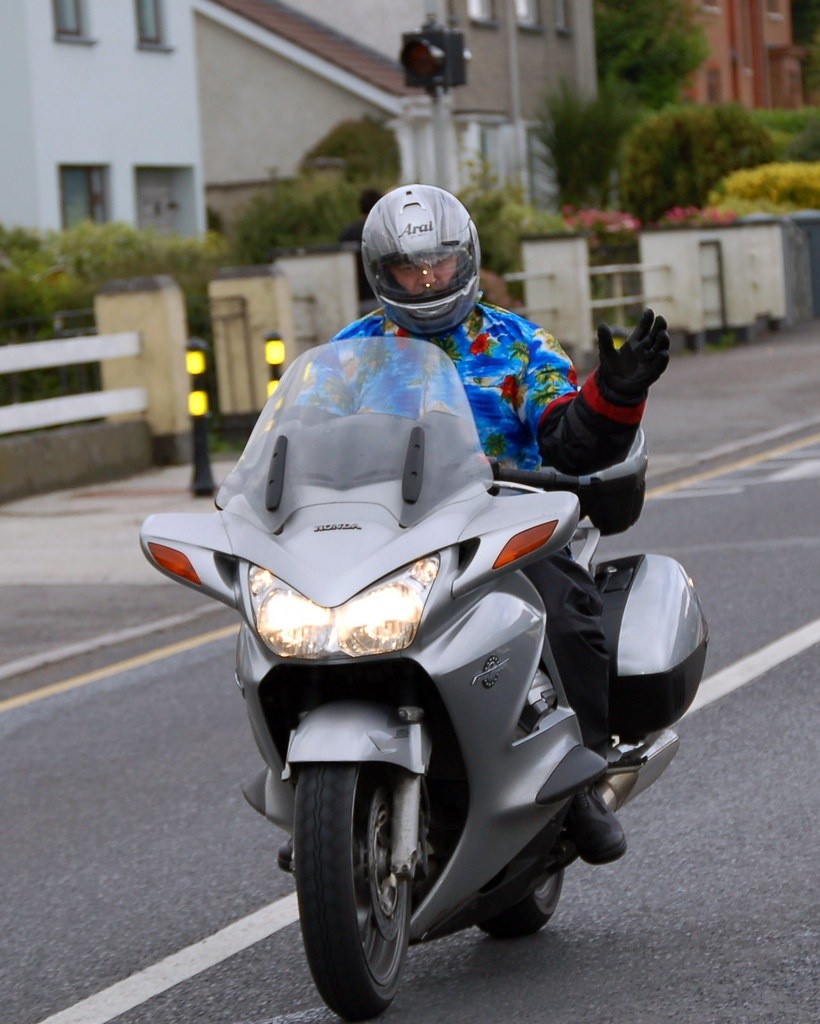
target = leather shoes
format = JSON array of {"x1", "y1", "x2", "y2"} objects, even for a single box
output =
[
  {"x1": 277, "y1": 838, "x2": 293, "y2": 872},
  {"x1": 562, "y1": 787, "x2": 627, "y2": 865}
]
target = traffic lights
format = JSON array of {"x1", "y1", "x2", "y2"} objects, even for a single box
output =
[{"x1": 401, "y1": 30, "x2": 449, "y2": 89}]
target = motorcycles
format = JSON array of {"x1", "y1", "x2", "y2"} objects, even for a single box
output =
[{"x1": 138, "y1": 332, "x2": 711, "y2": 1024}]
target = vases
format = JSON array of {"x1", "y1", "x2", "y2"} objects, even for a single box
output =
[{"x1": 597, "y1": 234, "x2": 641, "y2": 281}]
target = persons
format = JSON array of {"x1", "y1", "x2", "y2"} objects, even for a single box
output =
[{"x1": 275, "y1": 183, "x2": 669, "y2": 873}]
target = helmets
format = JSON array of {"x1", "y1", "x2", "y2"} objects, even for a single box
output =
[{"x1": 361, "y1": 185, "x2": 481, "y2": 336}]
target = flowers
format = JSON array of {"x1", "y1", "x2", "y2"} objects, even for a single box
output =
[{"x1": 565, "y1": 203, "x2": 735, "y2": 246}]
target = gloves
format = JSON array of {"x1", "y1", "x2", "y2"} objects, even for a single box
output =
[{"x1": 597, "y1": 307, "x2": 670, "y2": 408}]
